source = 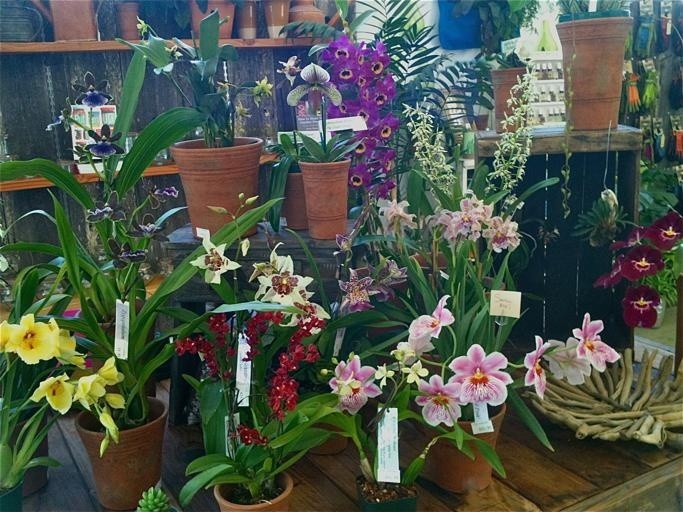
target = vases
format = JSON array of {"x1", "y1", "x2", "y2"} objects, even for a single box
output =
[
  {"x1": 355, "y1": 476, "x2": 419, "y2": 512},
  {"x1": 422, "y1": 402, "x2": 507, "y2": 495},
  {"x1": 73, "y1": 397, "x2": 169, "y2": 511},
  {"x1": 1, "y1": 480, "x2": 24, "y2": 512},
  {"x1": 209, "y1": 476, "x2": 295, "y2": 512}
]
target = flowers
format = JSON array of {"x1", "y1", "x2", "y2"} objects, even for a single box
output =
[{"x1": 0, "y1": 0, "x2": 683, "y2": 484}]
